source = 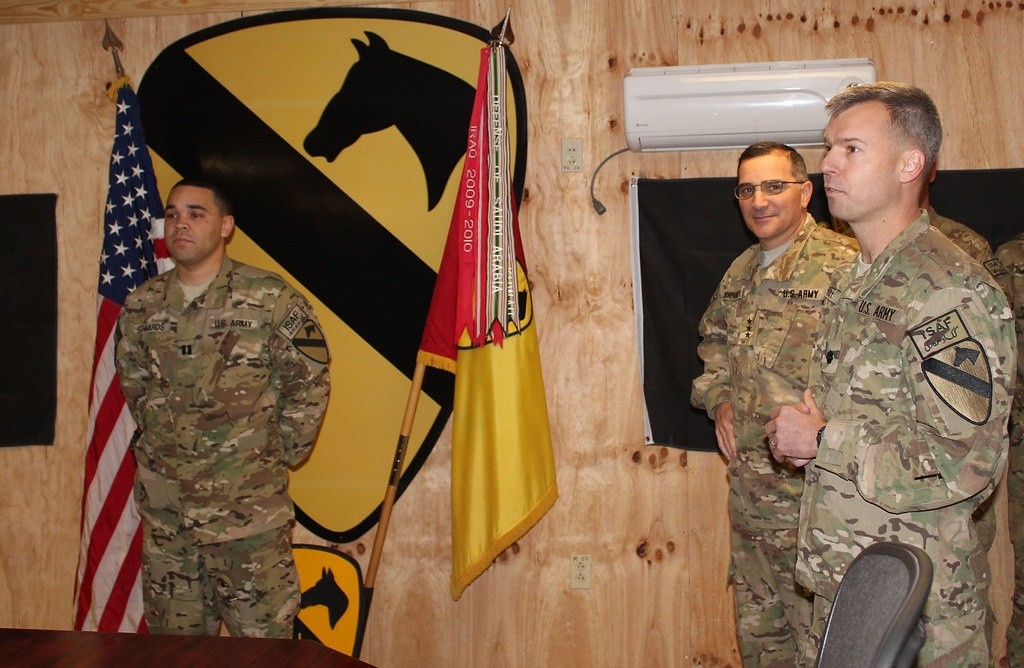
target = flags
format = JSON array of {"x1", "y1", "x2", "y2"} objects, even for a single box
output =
[
  {"x1": 71, "y1": 85, "x2": 175, "y2": 635},
  {"x1": 418, "y1": 45, "x2": 559, "y2": 597}
]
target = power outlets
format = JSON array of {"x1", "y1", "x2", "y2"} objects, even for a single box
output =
[{"x1": 560, "y1": 136, "x2": 584, "y2": 174}]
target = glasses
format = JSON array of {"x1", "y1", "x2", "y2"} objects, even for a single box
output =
[{"x1": 734, "y1": 180, "x2": 805, "y2": 200}]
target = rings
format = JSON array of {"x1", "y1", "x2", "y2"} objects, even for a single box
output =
[{"x1": 770, "y1": 441, "x2": 776, "y2": 446}]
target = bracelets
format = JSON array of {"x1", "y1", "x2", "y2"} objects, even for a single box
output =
[{"x1": 817, "y1": 426, "x2": 826, "y2": 445}]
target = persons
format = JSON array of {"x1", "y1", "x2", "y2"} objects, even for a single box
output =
[
  {"x1": 764, "y1": 81, "x2": 1016, "y2": 668},
  {"x1": 693, "y1": 139, "x2": 863, "y2": 668},
  {"x1": 924, "y1": 165, "x2": 1024, "y2": 668},
  {"x1": 114, "y1": 175, "x2": 331, "y2": 639}
]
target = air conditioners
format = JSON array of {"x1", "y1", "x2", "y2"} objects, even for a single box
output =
[{"x1": 621, "y1": 57, "x2": 878, "y2": 153}]
